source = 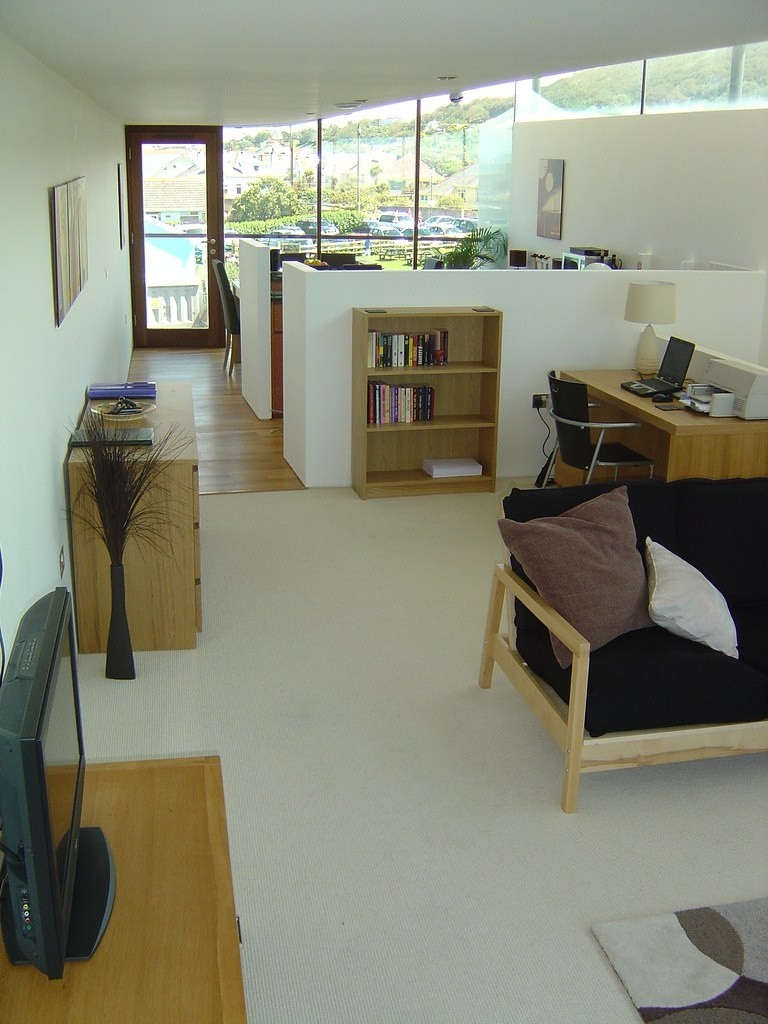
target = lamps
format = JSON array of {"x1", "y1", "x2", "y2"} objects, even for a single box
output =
[
  {"x1": 624, "y1": 280, "x2": 676, "y2": 375},
  {"x1": 509, "y1": 249, "x2": 526, "y2": 270}
]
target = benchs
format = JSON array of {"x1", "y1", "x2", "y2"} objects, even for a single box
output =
[{"x1": 479, "y1": 474, "x2": 768, "y2": 813}]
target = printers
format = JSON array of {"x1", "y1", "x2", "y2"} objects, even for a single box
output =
[{"x1": 678, "y1": 359, "x2": 768, "y2": 420}]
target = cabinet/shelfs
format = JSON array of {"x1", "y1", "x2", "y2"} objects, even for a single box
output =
[
  {"x1": 351, "y1": 307, "x2": 503, "y2": 500},
  {"x1": 270, "y1": 276, "x2": 283, "y2": 417},
  {"x1": 0, "y1": 752, "x2": 249, "y2": 1023},
  {"x1": 68, "y1": 381, "x2": 201, "y2": 655}
]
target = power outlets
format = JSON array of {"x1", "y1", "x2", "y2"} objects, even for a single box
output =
[
  {"x1": 58, "y1": 546, "x2": 66, "y2": 580},
  {"x1": 533, "y1": 394, "x2": 548, "y2": 408}
]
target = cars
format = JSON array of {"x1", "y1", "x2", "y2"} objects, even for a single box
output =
[
  {"x1": 256, "y1": 225, "x2": 313, "y2": 251},
  {"x1": 296, "y1": 219, "x2": 340, "y2": 235},
  {"x1": 182, "y1": 223, "x2": 235, "y2": 263},
  {"x1": 353, "y1": 212, "x2": 478, "y2": 237}
]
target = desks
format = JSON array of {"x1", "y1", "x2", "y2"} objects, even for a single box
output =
[
  {"x1": 554, "y1": 368, "x2": 767, "y2": 488},
  {"x1": 231, "y1": 280, "x2": 241, "y2": 365}
]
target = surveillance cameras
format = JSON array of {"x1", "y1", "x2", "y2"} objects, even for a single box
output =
[{"x1": 449, "y1": 92, "x2": 463, "y2": 103}]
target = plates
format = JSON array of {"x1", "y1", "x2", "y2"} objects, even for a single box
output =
[{"x1": 90, "y1": 400, "x2": 157, "y2": 422}]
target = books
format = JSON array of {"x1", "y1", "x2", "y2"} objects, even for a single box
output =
[
  {"x1": 73, "y1": 429, "x2": 155, "y2": 445},
  {"x1": 367, "y1": 328, "x2": 449, "y2": 368},
  {"x1": 367, "y1": 380, "x2": 436, "y2": 424}
]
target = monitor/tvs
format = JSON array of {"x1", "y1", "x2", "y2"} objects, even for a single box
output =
[
  {"x1": 508, "y1": 249, "x2": 528, "y2": 268},
  {"x1": 0, "y1": 587, "x2": 116, "y2": 980}
]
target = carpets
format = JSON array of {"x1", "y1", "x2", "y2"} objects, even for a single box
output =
[
  {"x1": 73, "y1": 485, "x2": 768, "y2": 1024},
  {"x1": 591, "y1": 898, "x2": 768, "y2": 1024}
]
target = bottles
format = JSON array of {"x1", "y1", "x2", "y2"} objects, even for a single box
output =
[
  {"x1": 599, "y1": 249, "x2": 605, "y2": 263},
  {"x1": 609, "y1": 254, "x2": 618, "y2": 270}
]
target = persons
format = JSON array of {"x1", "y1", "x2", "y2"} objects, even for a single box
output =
[{"x1": 363, "y1": 239, "x2": 372, "y2": 256}]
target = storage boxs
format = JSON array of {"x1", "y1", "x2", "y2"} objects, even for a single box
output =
[{"x1": 421, "y1": 458, "x2": 482, "y2": 478}]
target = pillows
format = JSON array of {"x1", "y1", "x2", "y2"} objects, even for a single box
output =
[
  {"x1": 497, "y1": 485, "x2": 654, "y2": 669},
  {"x1": 643, "y1": 536, "x2": 739, "y2": 659}
]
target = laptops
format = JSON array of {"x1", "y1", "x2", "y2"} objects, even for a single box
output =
[{"x1": 621, "y1": 336, "x2": 695, "y2": 397}]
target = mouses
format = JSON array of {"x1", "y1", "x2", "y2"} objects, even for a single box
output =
[{"x1": 652, "y1": 394, "x2": 673, "y2": 402}]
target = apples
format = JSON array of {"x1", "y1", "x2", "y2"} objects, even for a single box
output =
[{"x1": 304, "y1": 259, "x2": 328, "y2": 266}]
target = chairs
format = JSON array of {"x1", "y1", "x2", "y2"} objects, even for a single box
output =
[{"x1": 212, "y1": 259, "x2": 242, "y2": 377}]
